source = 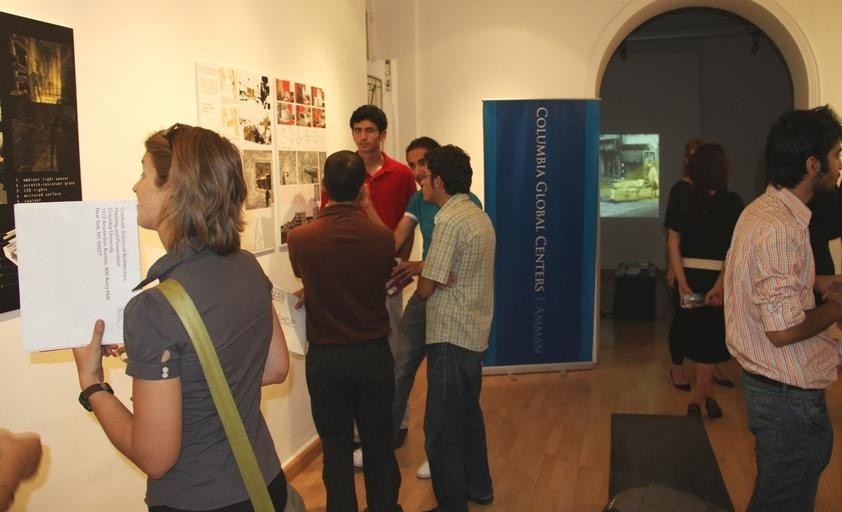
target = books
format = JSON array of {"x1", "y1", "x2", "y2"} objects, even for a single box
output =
[
  {"x1": 272, "y1": 284, "x2": 309, "y2": 358},
  {"x1": 14, "y1": 202, "x2": 143, "y2": 353}
]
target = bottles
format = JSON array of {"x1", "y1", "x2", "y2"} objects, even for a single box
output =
[{"x1": 679, "y1": 292, "x2": 708, "y2": 310}]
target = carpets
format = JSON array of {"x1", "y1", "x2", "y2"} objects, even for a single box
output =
[{"x1": 605, "y1": 411, "x2": 734, "y2": 511}]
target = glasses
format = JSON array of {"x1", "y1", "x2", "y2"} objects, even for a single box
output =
[{"x1": 160, "y1": 123, "x2": 181, "y2": 151}]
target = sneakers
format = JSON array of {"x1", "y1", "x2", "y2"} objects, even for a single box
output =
[
  {"x1": 688, "y1": 402, "x2": 701, "y2": 418},
  {"x1": 417, "y1": 457, "x2": 431, "y2": 479},
  {"x1": 706, "y1": 398, "x2": 722, "y2": 418},
  {"x1": 353, "y1": 446, "x2": 400, "y2": 467}
]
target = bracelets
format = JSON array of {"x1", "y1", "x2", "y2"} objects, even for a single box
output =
[
  {"x1": 0, "y1": 481, "x2": 15, "y2": 504},
  {"x1": 78, "y1": 380, "x2": 115, "y2": 413}
]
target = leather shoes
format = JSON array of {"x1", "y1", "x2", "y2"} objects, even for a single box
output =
[
  {"x1": 671, "y1": 368, "x2": 691, "y2": 390},
  {"x1": 713, "y1": 373, "x2": 734, "y2": 387}
]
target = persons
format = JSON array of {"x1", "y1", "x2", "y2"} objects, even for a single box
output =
[
  {"x1": 288, "y1": 105, "x2": 500, "y2": 512},
  {"x1": 72, "y1": 122, "x2": 305, "y2": 511},
  {"x1": 663, "y1": 140, "x2": 841, "y2": 421},
  {"x1": 721, "y1": 103, "x2": 841, "y2": 512},
  {"x1": 648, "y1": 161, "x2": 658, "y2": 199},
  {"x1": 0, "y1": 428, "x2": 43, "y2": 512}
]
